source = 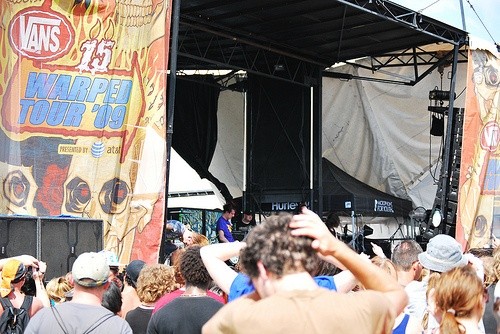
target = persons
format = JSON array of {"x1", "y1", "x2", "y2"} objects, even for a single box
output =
[
  {"x1": 236, "y1": 209, "x2": 256, "y2": 241},
  {"x1": 0, "y1": 234, "x2": 500, "y2": 334},
  {"x1": 326, "y1": 213, "x2": 340, "y2": 237},
  {"x1": 216, "y1": 205, "x2": 236, "y2": 244},
  {"x1": 201, "y1": 206, "x2": 409, "y2": 334}
]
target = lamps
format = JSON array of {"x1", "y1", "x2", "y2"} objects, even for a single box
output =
[
  {"x1": 429, "y1": 86, "x2": 456, "y2": 105},
  {"x1": 409, "y1": 206, "x2": 445, "y2": 243}
]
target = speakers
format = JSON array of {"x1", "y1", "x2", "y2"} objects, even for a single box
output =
[{"x1": 423, "y1": 107, "x2": 465, "y2": 242}]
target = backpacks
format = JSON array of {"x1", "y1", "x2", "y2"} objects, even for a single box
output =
[{"x1": 0, "y1": 295, "x2": 33, "y2": 334}]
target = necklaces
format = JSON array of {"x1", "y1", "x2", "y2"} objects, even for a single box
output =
[{"x1": 142, "y1": 304, "x2": 155, "y2": 308}]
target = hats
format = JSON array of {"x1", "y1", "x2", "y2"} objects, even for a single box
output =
[
  {"x1": 241, "y1": 208, "x2": 253, "y2": 215},
  {"x1": 221, "y1": 202, "x2": 237, "y2": 213},
  {"x1": 1, "y1": 258, "x2": 26, "y2": 284},
  {"x1": 99, "y1": 250, "x2": 120, "y2": 267},
  {"x1": 418, "y1": 234, "x2": 469, "y2": 272},
  {"x1": 72, "y1": 252, "x2": 110, "y2": 287},
  {"x1": 464, "y1": 253, "x2": 484, "y2": 286},
  {"x1": 126, "y1": 260, "x2": 147, "y2": 287}
]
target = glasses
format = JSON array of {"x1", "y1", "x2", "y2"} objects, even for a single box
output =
[{"x1": 481, "y1": 288, "x2": 489, "y2": 303}]
